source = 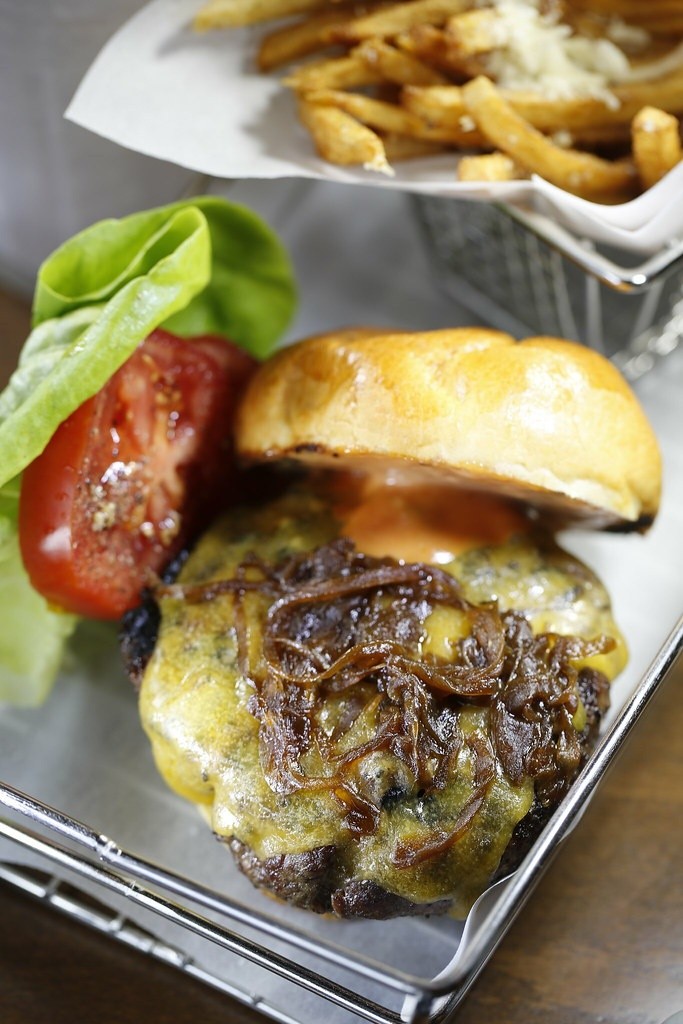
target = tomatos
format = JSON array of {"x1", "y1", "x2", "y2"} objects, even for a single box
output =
[{"x1": 20, "y1": 327, "x2": 265, "y2": 619}]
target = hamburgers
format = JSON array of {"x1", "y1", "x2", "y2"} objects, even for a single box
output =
[{"x1": 138, "y1": 322, "x2": 659, "y2": 923}]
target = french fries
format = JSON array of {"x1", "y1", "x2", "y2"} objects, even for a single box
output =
[{"x1": 188, "y1": 0, "x2": 683, "y2": 198}]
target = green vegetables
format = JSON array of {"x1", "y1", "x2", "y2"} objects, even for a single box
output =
[{"x1": 0, "y1": 196, "x2": 295, "y2": 710}]
